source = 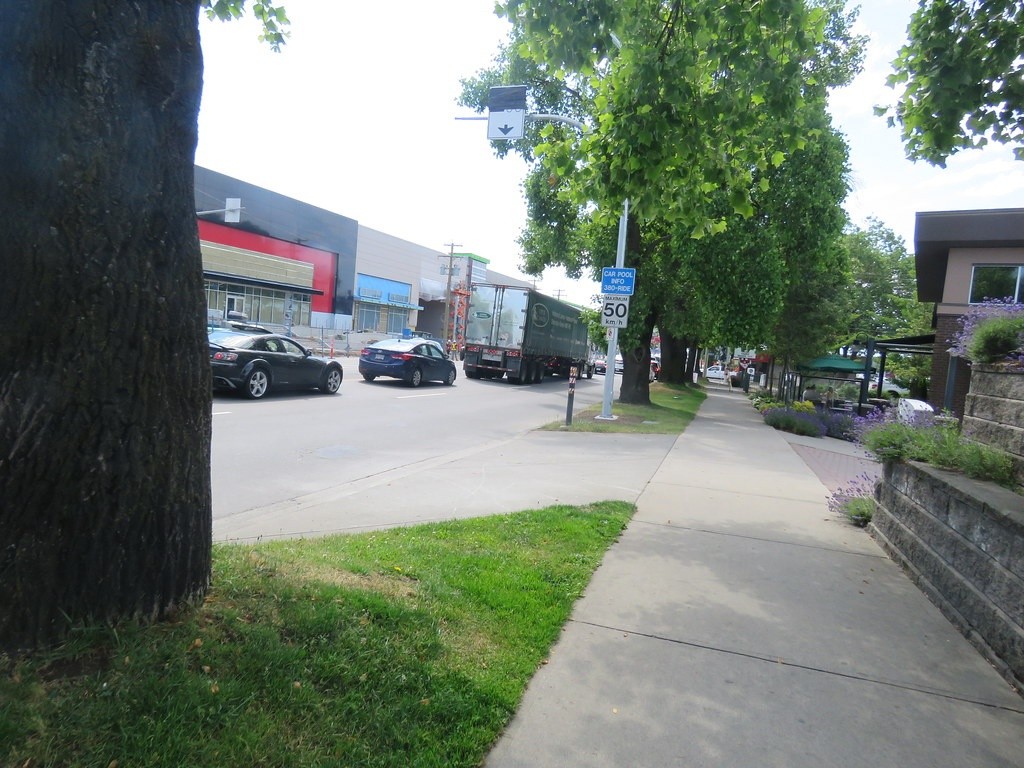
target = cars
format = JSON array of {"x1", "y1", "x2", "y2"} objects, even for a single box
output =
[
  {"x1": 208, "y1": 323, "x2": 344, "y2": 401},
  {"x1": 594, "y1": 349, "x2": 662, "y2": 382},
  {"x1": 856, "y1": 373, "x2": 910, "y2": 399},
  {"x1": 358, "y1": 337, "x2": 457, "y2": 388}
]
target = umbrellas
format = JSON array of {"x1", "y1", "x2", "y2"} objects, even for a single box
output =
[{"x1": 794, "y1": 353, "x2": 877, "y2": 407}]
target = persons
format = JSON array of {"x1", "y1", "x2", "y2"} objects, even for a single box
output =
[{"x1": 803, "y1": 384, "x2": 839, "y2": 407}]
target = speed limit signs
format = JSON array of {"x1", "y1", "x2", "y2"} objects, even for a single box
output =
[{"x1": 600, "y1": 293, "x2": 630, "y2": 329}]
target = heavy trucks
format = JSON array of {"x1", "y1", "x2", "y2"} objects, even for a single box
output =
[{"x1": 459, "y1": 281, "x2": 595, "y2": 386}]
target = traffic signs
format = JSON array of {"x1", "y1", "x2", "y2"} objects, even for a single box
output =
[{"x1": 486, "y1": 83, "x2": 526, "y2": 140}]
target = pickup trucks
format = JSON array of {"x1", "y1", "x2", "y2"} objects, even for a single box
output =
[{"x1": 706, "y1": 365, "x2": 739, "y2": 380}]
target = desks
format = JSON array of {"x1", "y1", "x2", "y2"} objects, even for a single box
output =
[
  {"x1": 870, "y1": 399, "x2": 890, "y2": 410},
  {"x1": 853, "y1": 403, "x2": 876, "y2": 418}
]
